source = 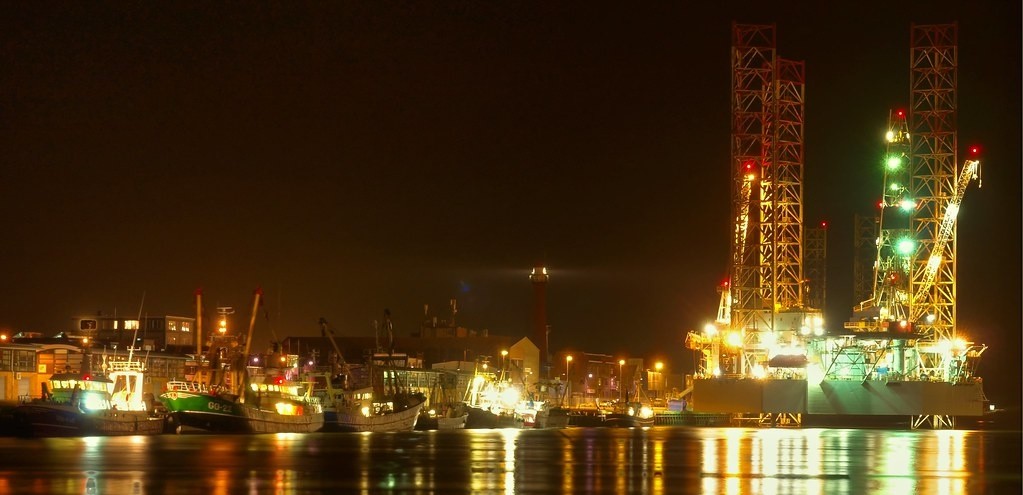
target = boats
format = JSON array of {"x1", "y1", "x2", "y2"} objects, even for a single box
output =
[
  {"x1": 0, "y1": 294, "x2": 166, "y2": 435},
  {"x1": 158, "y1": 290, "x2": 324, "y2": 437},
  {"x1": 311, "y1": 311, "x2": 658, "y2": 435}
]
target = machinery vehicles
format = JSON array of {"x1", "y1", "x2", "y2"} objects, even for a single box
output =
[
  {"x1": 872, "y1": 111, "x2": 984, "y2": 325},
  {"x1": 685, "y1": 163, "x2": 755, "y2": 377}
]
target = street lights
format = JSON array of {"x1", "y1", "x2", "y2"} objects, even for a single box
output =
[
  {"x1": 499, "y1": 349, "x2": 511, "y2": 379},
  {"x1": 566, "y1": 354, "x2": 573, "y2": 387},
  {"x1": 618, "y1": 359, "x2": 626, "y2": 401}
]
